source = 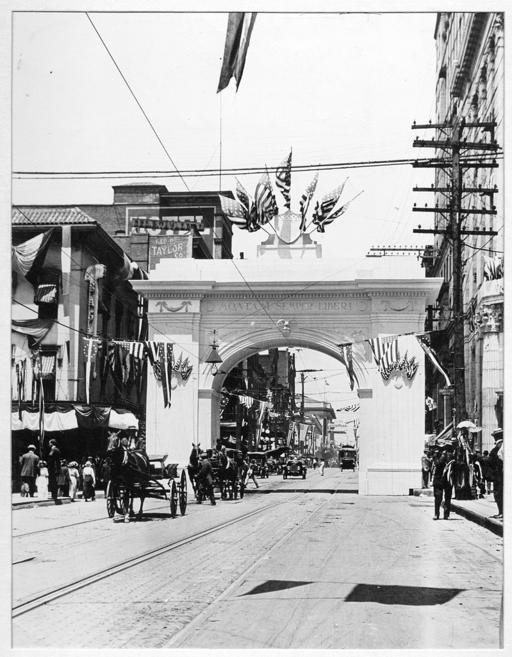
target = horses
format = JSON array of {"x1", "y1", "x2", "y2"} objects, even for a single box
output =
[
  {"x1": 107, "y1": 430, "x2": 151, "y2": 517},
  {"x1": 188, "y1": 443, "x2": 238, "y2": 500}
]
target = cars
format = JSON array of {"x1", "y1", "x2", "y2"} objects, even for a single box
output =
[{"x1": 282, "y1": 459, "x2": 307, "y2": 479}]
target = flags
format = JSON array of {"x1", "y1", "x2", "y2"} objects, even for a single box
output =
[
  {"x1": 254, "y1": 167, "x2": 279, "y2": 225},
  {"x1": 231, "y1": 177, "x2": 257, "y2": 232},
  {"x1": 276, "y1": 153, "x2": 292, "y2": 209},
  {"x1": 339, "y1": 334, "x2": 399, "y2": 391},
  {"x1": 81, "y1": 337, "x2": 176, "y2": 409},
  {"x1": 316, "y1": 195, "x2": 361, "y2": 233},
  {"x1": 312, "y1": 181, "x2": 346, "y2": 225},
  {"x1": 299, "y1": 170, "x2": 321, "y2": 232},
  {"x1": 219, "y1": 194, "x2": 260, "y2": 232}
]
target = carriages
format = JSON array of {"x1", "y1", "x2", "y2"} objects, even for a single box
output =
[
  {"x1": 106, "y1": 430, "x2": 187, "y2": 523},
  {"x1": 184, "y1": 442, "x2": 247, "y2": 500}
]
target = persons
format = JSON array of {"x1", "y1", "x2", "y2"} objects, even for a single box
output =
[{"x1": 418, "y1": 427, "x2": 503, "y2": 521}]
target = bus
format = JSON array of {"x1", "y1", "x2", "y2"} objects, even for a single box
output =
[{"x1": 338, "y1": 445, "x2": 356, "y2": 468}]
